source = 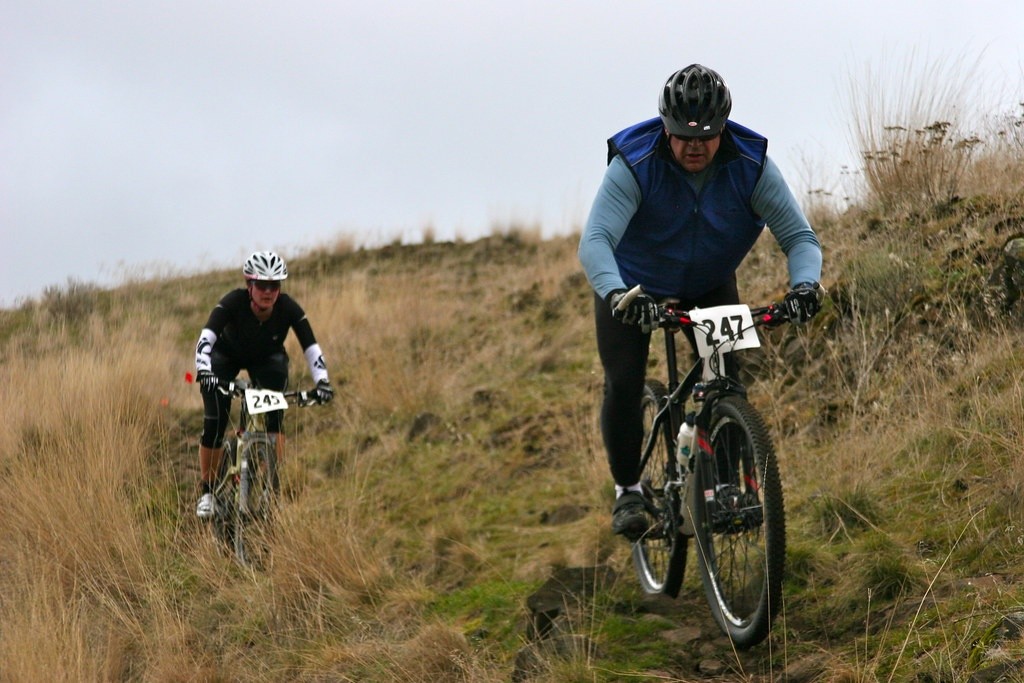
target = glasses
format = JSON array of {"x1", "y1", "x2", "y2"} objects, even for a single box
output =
[
  {"x1": 252, "y1": 281, "x2": 281, "y2": 291},
  {"x1": 671, "y1": 132, "x2": 720, "y2": 141}
]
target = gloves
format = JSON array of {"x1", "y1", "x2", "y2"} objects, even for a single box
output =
[
  {"x1": 195, "y1": 372, "x2": 219, "y2": 393},
  {"x1": 604, "y1": 287, "x2": 659, "y2": 333},
  {"x1": 310, "y1": 379, "x2": 334, "y2": 403},
  {"x1": 783, "y1": 282, "x2": 817, "y2": 324}
]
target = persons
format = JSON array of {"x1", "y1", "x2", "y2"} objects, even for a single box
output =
[
  {"x1": 578, "y1": 63, "x2": 825, "y2": 536},
  {"x1": 194, "y1": 251, "x2": 334, "y2": 516}
]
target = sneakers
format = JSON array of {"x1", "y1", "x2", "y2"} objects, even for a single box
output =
[
  {"x1": 195, "y1": 490, "x2": 215, "y2": 516},
  {"x1": 706, "y1": 486, "x2": 745, "y2": 523},
  {"x1": 609, "y1": 490, "x2": 650, "y2": 536}
]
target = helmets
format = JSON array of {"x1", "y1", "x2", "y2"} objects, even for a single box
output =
[
  {"x1": 658, "y1": 64, "x2": 732, "y2": 137},
  {"x1": 243, "y1": 251, "x2": 288, "y2": 281}
]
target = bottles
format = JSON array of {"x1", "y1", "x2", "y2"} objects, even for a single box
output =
[
  {"x1": 675, "y1": 411, "x2": 697, "y2": 466},
  {"x1": 230, "y1": 428, "x2": 245, "y2": 474}
]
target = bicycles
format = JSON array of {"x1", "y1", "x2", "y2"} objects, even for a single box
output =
[
  {"x1": 628, "y1": 302, "x2": 788, "y2": 650},
  {"x1": 212, "y1": 373, "x2": 318, "y2": 574}
]
